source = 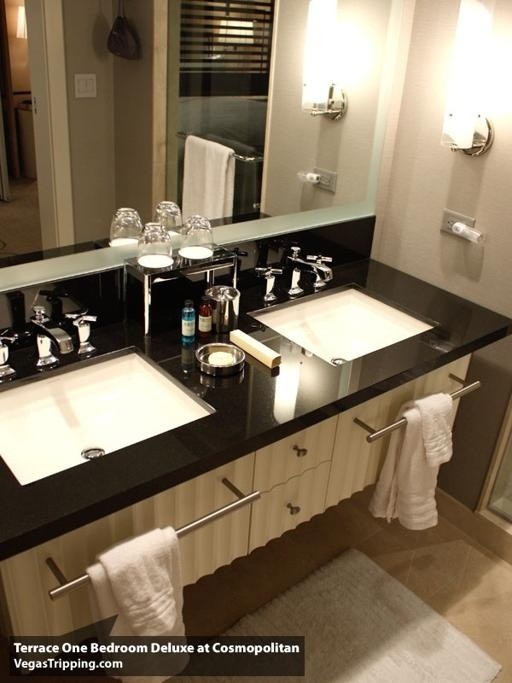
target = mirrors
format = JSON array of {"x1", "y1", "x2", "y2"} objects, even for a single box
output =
[{"x1": 0, "y1": 1, "x2": 395, "y2": 268}]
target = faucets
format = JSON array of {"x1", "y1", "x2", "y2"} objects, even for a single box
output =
[
  {"x1": 3, "y1": 290, "x2": 32, "y2": 343},
  {"x1": 27, "y1": 305, "x2": 73, "y2": 368},
  {"x1": 284, "y1": 245, "x2": 334, "y2": 298}
]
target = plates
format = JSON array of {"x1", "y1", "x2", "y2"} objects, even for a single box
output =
[{"x1": 194, "y1": 341, "x2": 247, "y2": 377}]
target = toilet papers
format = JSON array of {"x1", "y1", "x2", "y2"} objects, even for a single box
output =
[{"x1": 207, "y1": 349, "x2": 237, "y2": 368}]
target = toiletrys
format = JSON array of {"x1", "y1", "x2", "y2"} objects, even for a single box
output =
[
  {"x1": 181, "y1": 300, "x2": 197, "y2": 338},
  {"x1": 181, "y1": 336, "x2": 195, "y2": 375}
]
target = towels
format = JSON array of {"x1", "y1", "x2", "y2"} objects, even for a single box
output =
[
  {"x1": 97, "y1": 527, "x2": 177, "y2": 638},
  {"x1": 369, "y1": 406, "x2": 463, "y2": 541},
  {"x1": 181, "y1": 134, "x2": 236, "y2": 221},
  {"x1": 85, "y1": 526, "x2": 190, "y2": 683},
  {"x1": 397, "y1": 393, "x2": 453, "y2": 465}
]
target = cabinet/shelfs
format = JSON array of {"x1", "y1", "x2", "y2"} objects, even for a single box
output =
[{"x1": 250, "y1": 416, "x2": 341, "y2": 553}]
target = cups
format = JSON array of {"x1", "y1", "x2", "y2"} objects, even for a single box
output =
[
  {"x1": 109, "y1": 207, "x2": 142, "y2": 244},
  {"x1": 179, "y1": 215, "x2": 214, "y2": 258},
  {"x1": 137, "y1": 222, "x2": 172, "y2": 267},
  {"x1": 206, "y1": 284, "x2": 241, "y2": 333},
  {"x1": 152, "y1": 201, "x2": 182, "y2": 238}
]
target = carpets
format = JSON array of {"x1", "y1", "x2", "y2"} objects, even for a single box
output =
[{"x1": 216, "y1": 545, "x2": 502, "y2": 682}]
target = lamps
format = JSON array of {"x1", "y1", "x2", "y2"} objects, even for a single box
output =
[
  {"x1": 438, "y1": 101, "x2": 496, "y2": 159},
  {"x1": 298, "y1": 66, "x2": 351, "y2": 121},
  {"x1": 18, "y1": 5, "x2": 29, "y2": 40},
  {"x1": 212, "y1": 20, "x2": 257, "y2": 52}
]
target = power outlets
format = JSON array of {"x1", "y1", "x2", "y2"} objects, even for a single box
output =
[
  {"x1": 308, "y1": 168, "x2": 337, "y2": 191},
  {"x1": 438, "y1": 208, "x2": 478, "y2": 238}
]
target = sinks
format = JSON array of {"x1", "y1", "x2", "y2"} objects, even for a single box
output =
[
  {"x1": 0, "y1": 344, "x2": 219, "y2": 489},
  {"x1": 246, "y1": 281, "x2": 436, "y2": 369}
]
